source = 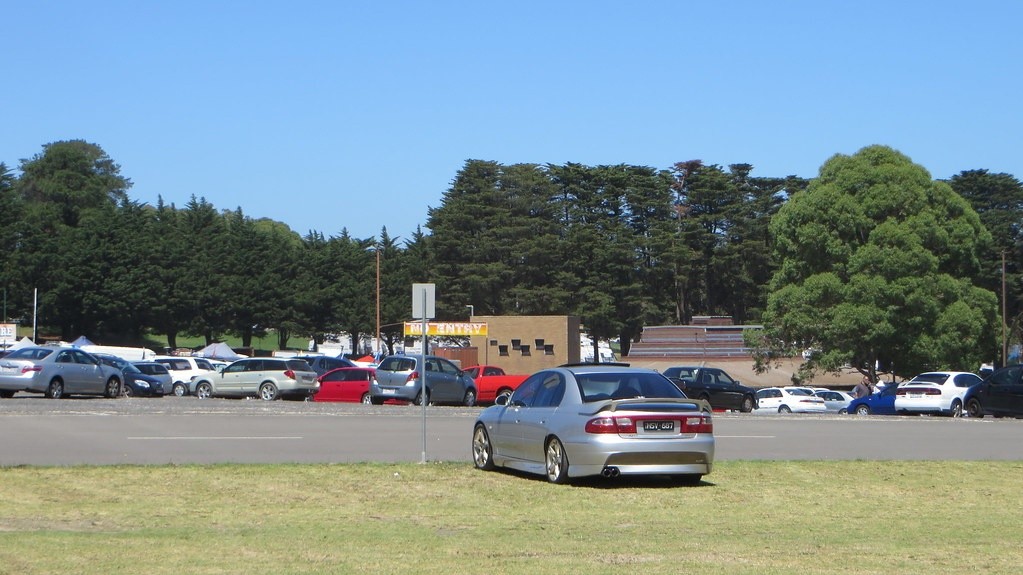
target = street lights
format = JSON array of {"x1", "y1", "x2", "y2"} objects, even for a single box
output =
[
  {"x1": 364, "y1": 243, "x2": 386, "y2": 353},
  {"x1": 995, "y1": 246, "x2": 1013, "y2": 367}
]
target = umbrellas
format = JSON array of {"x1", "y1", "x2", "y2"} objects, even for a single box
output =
[
  {"x1": 6, "y1": 337, "x2": 41, "y2": 351},
  {"x1": 71, "y1": 336, "x2": 96, "y2": 347}
]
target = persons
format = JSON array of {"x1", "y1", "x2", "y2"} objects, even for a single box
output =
[
  {"x1": 855, "y1": 376, "x2": 873, "y2": 398},
  {"x1": 980, "y1": 359, "x2": 994, "y2": 379}
]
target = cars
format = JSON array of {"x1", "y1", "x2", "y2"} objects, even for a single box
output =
[
  {"x1": 724, "y1": 385, "x2": 826, "y2": 415},
  {"x1": 369, "y1": 354, "x2": 477, "y2": 408},
  {"x1": 472, "y1": 361, "x2": 716, "y2": 485},
  {"x1": 962, "y1": 364, "x2": 1023, "y2": 419},
  {"x1": 662, "y1": 366, "x2": 759, "y2": 413},
  {"x1": 306, "y1": 366, "x2": 411, "y2": 406},
  {"x1": 0, "y1": 346, "x2": 124, "y2": 399},
  {"x1": 88, "y1": 353, "x2": 165, "y2": 399},
  {"x1": 783, "y1": 386, "x2": 831, "y2": 397},
  {"x1": 79, "y1": 346, "x2": 249, "y2": 397},
  {"x1": 894, "y1": 371, "x2": 984, "y2": 418},
  {"x1": 847, "y1": 382, "x2": 899, "y2": 415},
  {"x1": 808, "y1": 390, "x2": 855, "y2": 415}
]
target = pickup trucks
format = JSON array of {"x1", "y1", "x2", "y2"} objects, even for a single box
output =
[{"x1": 461, "y1": 365, "x2": 531, "y2": 406}]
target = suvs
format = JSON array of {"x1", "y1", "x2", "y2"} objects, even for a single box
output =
[{"x1": 190, "y1": 356, "x2": 320, "y2": 402}]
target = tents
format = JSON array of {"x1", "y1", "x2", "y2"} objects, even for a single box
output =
[{"x1": 192, "y1": 342, "x2": 243, "y2": 362}]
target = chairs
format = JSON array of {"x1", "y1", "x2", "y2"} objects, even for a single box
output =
[
  {"x1": 611, "y1": 387, "x2": 640, "y2": 397},
  {"x1": 37, "y1": 353, "x2": 46, "y2": 359},
  {"x1": 425, "y1": 362, "x2": 433, "y2": 371},
  {"x1": 703, "y1": 375, "x2": 712, "y2": 383},
  {"x1": 199, "y1": 364, "x2": 207, "y2": 369}
]
